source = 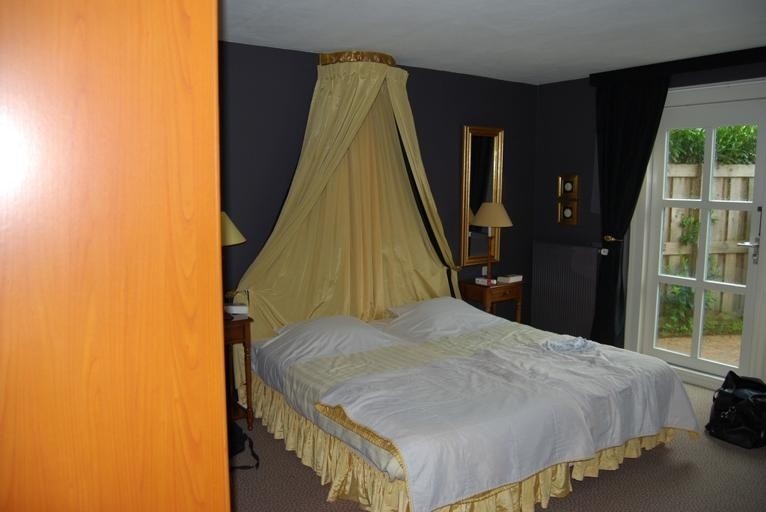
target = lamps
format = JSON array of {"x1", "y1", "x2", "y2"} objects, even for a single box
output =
[
  {"x1": 221, "y1": 212, "x2": 248, "y2": 247},
  {"x1": 470, "y1": 201, "x2": 513, "y2": 278}
]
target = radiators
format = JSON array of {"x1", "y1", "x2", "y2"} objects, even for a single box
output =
[{"x1": 528, "y1": 239, "x2": 602, "y2": 340}]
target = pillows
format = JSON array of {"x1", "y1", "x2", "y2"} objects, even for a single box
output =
[{"x1": 277, "y1": 297, "x2": 482, "y2": 356}]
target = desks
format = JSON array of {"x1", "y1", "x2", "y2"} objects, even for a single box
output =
[
  {"x1": 223, "y1": 314, "x2": 255, "y2": 432},
  {"x1": 459, "y1": 277, "x2": 526, "y2": 323}
]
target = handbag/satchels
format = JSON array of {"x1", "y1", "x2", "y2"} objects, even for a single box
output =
[
  {"x1": 705, "y1": 371, "x2": 766, "y2": 448},
  {"x1": 228, "y1": 421, "x2": 244, "y2": 457}
]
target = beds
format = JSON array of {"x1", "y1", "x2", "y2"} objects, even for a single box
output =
[{"x1": 253, "y1": 296, "x2": 692, "y2": 512}]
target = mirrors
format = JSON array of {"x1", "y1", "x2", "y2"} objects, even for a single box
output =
[{"x1": 459, "y1": 125, "x2": 504, "y2": 267}]
